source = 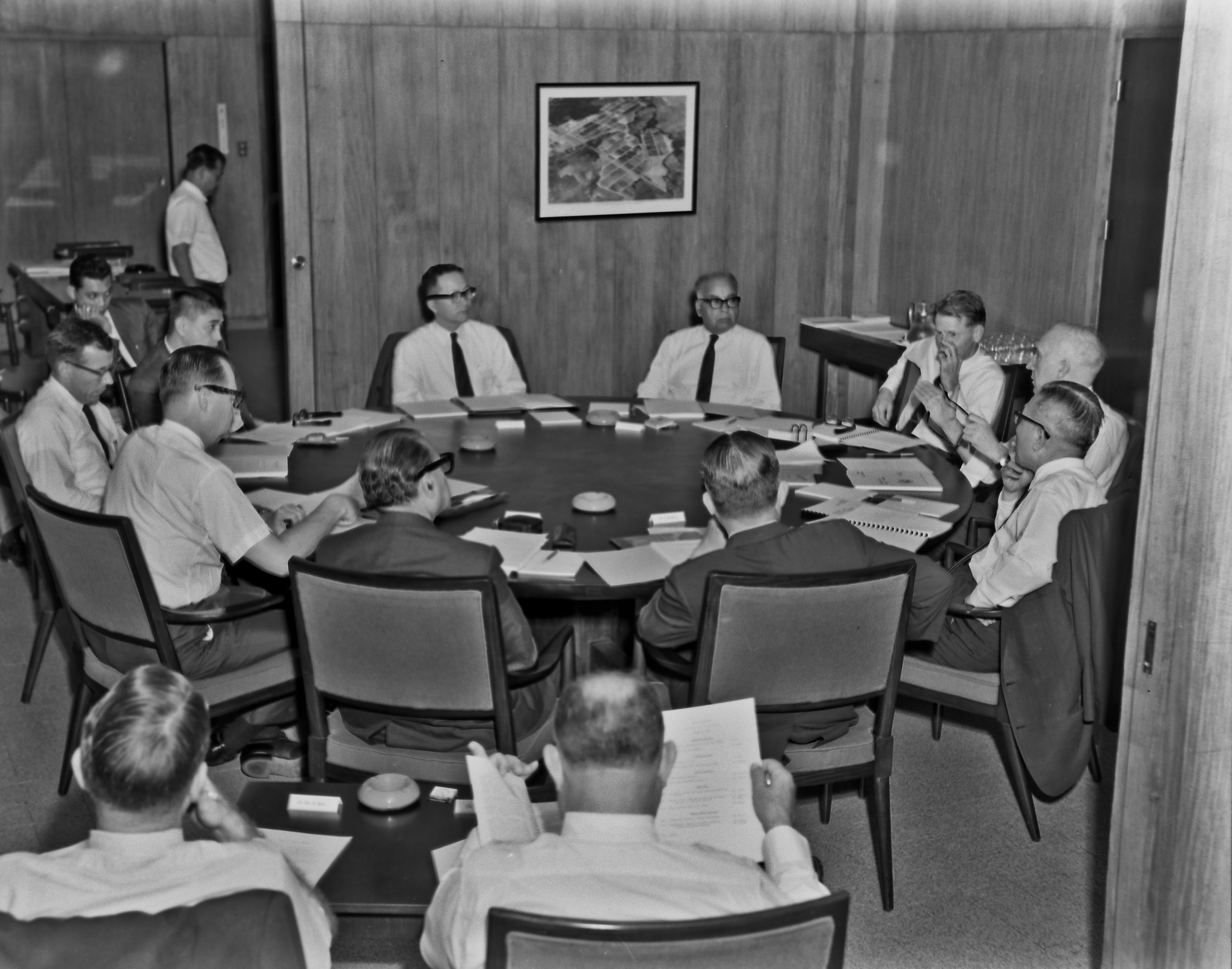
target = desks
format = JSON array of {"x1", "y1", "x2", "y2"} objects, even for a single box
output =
[
  {"x1": 210, "y1": 396, "x2": 973, "y2": 600},
  {"x1": 235, "y1": 780, "x2": 558, "y2": 969},
  {"x1": 799, "y1": 324, "x2": 1033, "y2": 420}
]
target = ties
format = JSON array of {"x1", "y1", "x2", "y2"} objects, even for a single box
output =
[
  {"x1": 900, "y1": 376, "x2": 941, "y2": 435},
  {"x1": 948, "y1": 490, "x2": 1028, "y2": 573},
  {"x1": 449, "y1": 333, "x2": 475, "y2": 397},
  {"x1": 696, "y1": 334, "x2": 718, "y2": 403},
  {"x1": 82, "y1": 404, "x2": 115, "y2": 469}
]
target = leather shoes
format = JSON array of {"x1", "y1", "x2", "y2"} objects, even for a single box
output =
[
  {"x1": 240, "y1": 737, "x2": 303, "y2": 779},
  {"x1": 205, "y1": 730, "x2": 231, "y2": 766}
]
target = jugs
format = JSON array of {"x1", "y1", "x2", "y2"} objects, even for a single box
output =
[{"x1": 906, "y1": 300, "x2": 936, "y2": 345}]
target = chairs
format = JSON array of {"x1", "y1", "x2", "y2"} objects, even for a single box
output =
[
  {"x1": 691, "y1": 559, "x2": 917, "y2": 912},
  {"x1": 486, "y1": 890, "x2": 851, "y2": 969},
  {"x1": 899, "y1": 499, "x2": 1129, "y2": 840},
  {"x1": 25, "y1": 486, "x2": 296, "y2": 796},
  {"x1": 114, "y1": 368, "x2": 136, "y2": 432},
  {"x1": 286, "y1": 555, "x2": 576, "y2": 786},
  {"x1": 0, "y1": 408, "x2": 54, "y2": 704}
]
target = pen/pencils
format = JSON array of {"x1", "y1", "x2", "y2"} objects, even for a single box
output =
[
  {"x1": 545, "y1": 549, "x2": 559, "y2": 562},
  {"x1": 865, "y1": 453, "x2": 915, "y2": 457},
  {"x1": 285, "y1": 504, "x2": 302, "y2": 529},
  {"x1": 868, "y1": 495, "x2": 917, "y2": 504},
  {"x1": 765, "y1": 771, "x2": 771, "y2": 786},
  {"x1": 722, "y1": 418, "x2": 737, "y2": 426}
]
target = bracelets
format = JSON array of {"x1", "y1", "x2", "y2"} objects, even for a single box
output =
[{"x1": 996, "y1": 453, "x2": 1010, "y2": 469}]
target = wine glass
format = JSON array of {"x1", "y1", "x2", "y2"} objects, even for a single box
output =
[{"x1": 979, "y1": 332, "x2": 1038, "y2": 364}]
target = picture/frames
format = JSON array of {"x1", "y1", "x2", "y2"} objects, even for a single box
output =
[{"x1": 536, "y1": 81, "x2": 700, "y2": 223}]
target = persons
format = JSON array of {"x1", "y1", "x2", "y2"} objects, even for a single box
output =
[
  {"x1": 310, "y1": 425, "x2": 561, "y2": 753},
  {"x1": 418, "y1": 668, "x2": 832, "y2": 969},
  {"x1": 914, "y1": 323, "x2": 1127, "y2": 558},
  {"x1": 63, "y1": 253, "x2": 164, "y2": 370},
  {"x1": 166, "y1": 142, "x2": 230, "y2": 300},
  {"x1": 857, "y1": 289, "x2": 1008, "y2": 499},
  {"x1": 0, "y1": 662, "x2": 338, "y2": 969},
  {"x1": 905, "y1": 379, "x2": 1106, "y2": 676},
  {"x1": 126, "y1": 286, "x2": 255, "y2": 440},
  {"x1": 14, "y1": 316, "x2": 128, "y2": 515},
  {"x1": 392, "y1": 262, "x2": 527, "y2": 410},
  {"x1": 638, "y1": 272, "x2": 782, "y2": 414},
  {"x1": 105, "y1": 343, "x2": 362, "y2": 783},
  {"x1": 636, "y1": 433, "x2": 953, "y2": 768}
]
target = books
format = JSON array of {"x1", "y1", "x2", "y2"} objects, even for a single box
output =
[
  {"x1": 644, "y1": 397, "x2": 761, "y2": 419},
  {"x1": 802, "y1": 456, "x2": 960, "y2": 553},
  {"x1": 209, "y1": 445, "x2": 293, "y2": 477},
  {"x1": 812, "y1": 420, "x2": 921, "y2": 452},
  {"x1": 396, "y1": 393, "x2": 579, "y2": 419}
]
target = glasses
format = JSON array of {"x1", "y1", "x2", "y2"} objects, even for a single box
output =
[
  {"x1": 413, "y1": 452, "x2": 455, "y2": 482},
  {"x1": 426, "y1": 285, "x2": 476, "y2": 302},
  {"x1": 290, "y1": 408, "x2": 343, "y2": 426},
  {"x1": 812, "y1": 416, "x2": 856, "y2": 435},
  {"x1": 64, "y1": 359, "x2": 116, "y2": 378},
  {"x1": 1013, "y1": 410, "x2": 1051, "y2": 440},
  {"x1": 195, "y1": 382, "x2": 246, "y2": 407},
  {"x1": 699, "y1": 296, "x2": 742, "y2": 309}
]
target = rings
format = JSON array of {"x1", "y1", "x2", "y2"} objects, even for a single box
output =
[
  {"x1": 946, "y1": 354, "x2": 952, "y2": 357},
  {"x1": 86, "y1": 308, "x2": 90, "y2": 312}
]
